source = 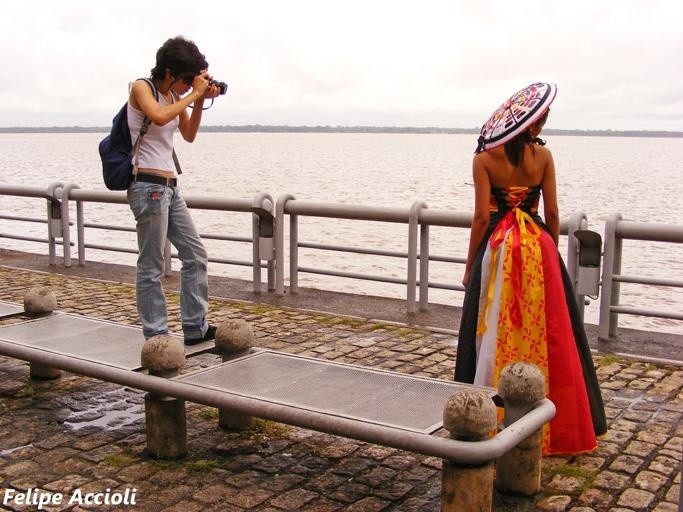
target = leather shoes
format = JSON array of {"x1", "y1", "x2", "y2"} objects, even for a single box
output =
[{"x1": 184, "y1": 325, "x2": 217, "y2": 345}]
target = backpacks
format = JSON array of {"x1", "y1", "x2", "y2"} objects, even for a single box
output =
[{"x1": 100, "y1": 78, "x2": 159, "y2": 190}]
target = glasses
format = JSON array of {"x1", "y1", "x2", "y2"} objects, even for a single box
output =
[{"x1": 169, "y1": 73, "x2": 194, "y2": 85}]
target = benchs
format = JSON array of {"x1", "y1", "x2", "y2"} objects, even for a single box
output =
[{"x1": 0, "y1": 285, "x2": 557, "y2": 512}]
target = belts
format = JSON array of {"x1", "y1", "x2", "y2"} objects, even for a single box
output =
[{"x1": 129, "y1": 175, "x2": 177, "y2": 187}]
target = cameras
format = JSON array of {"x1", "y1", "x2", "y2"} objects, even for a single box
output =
[{"x1": 205, "y1": 75, "x2": 228, "y2": 95}]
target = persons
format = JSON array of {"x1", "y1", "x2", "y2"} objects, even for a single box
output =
[
  {"x1": 126, "y1": 37, "x2": 221, "y2": 347},
  {"x1": 454, "y1": 82, "x2": 608, "y2": 456}
]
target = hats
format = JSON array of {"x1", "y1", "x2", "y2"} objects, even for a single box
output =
[{"x1": 480, "y1": 82, "x2": 558, "y2": 151}]
